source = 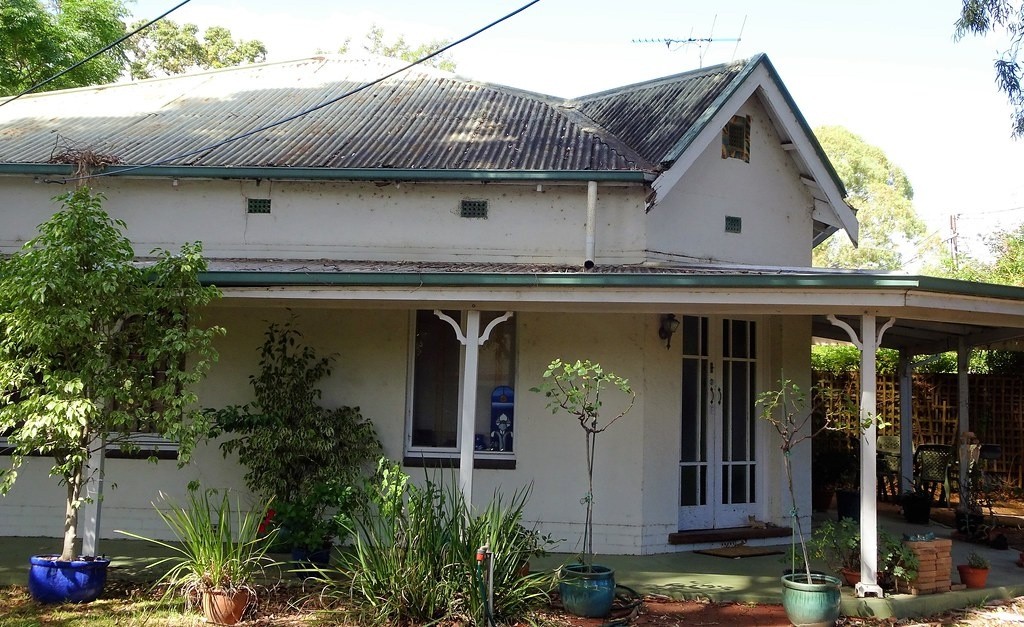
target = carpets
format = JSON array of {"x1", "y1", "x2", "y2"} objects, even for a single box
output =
[{"x1": 693, "y1": 546, "x2": 786, "y2": 559}]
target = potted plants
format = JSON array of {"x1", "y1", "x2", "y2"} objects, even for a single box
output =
[
  {"x1": 208, "y1": 308, "x2": 401, "y2": 581},
  {"x1": 956, "y1": 548, "x2": 991, "y2": 589},
  {"x1": 901, "y1": 475, "x2": 940, "y2": 525},
  {"x1": 0, "y1": 182, "x2": 228, "y2": 605},
  {"x1": 811, "y1": 516, "x2": 920, "y2": 586},
  {"x1": 834, "y1": 452, "x2": 861, "y2": 523},
  {"x1": 469, "y1": 508, "x2": 567, "y2": 587},
  {"x1": 950, "y1": 462, "x2": 1024, "y2": 550},
  {"x1": 754, "y1": 369, "x2": 892, "y2": 627},
  {"x1": 779, "y1": 538, "x2": 826, "y2": 576},
  {"x1": 528, "y1": 357, "x2": 636, "y2": 619},
  {"x1": 812, "y1": 452, "x2": 838, "y2": 513},
  {"x1": 113, "y1": 482, "x2": 284, "y2": 626}
]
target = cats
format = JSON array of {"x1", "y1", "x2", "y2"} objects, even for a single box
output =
[{"x1": 749, "y1": 515, "x2": 769, "y2": 529}]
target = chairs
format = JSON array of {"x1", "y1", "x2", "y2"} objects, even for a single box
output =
[
  {"x1": 875, "y1": 435, "x2": 914, "y2": 502},
  {"x1": 914, "y1": 443, "x2": 957, "y2": 507},
  {"x1": 978, "y1": 444, "x2": 1008, "y2": 506}
]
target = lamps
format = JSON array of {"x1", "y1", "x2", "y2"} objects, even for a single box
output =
[{"x1": 657, "y1": 314, "x2": 680, "y2": 352}]
